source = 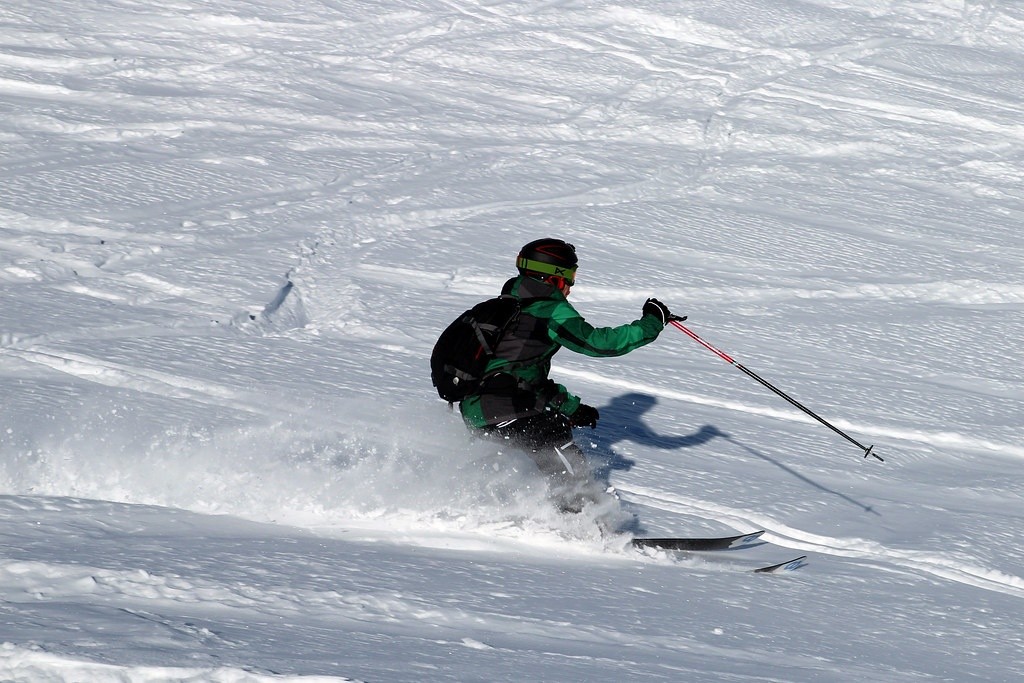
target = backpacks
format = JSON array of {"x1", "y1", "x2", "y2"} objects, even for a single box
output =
[{"x1": 431, "y1": 277, "x2": 565, "y2": 403}]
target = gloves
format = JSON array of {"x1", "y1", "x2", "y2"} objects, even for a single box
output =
[
  {"x1": 568, "y1": 403, "x2": 600, "y2": 430},
  {"x1": 643, "y1": 298, "x2": 670, "y2": 324}
]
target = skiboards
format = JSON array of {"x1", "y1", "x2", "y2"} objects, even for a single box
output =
[{"x1": 478, "y1": 515, "x2": 807, "y2": 574}]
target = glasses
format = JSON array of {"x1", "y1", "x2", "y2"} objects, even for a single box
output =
[{"x1": 516, "y1": 256, "x2": 579, "y2": 286}]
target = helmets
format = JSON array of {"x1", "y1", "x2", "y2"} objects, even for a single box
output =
[{"x1": 517, "y1": 238, "x2": 578, "y2": 291}]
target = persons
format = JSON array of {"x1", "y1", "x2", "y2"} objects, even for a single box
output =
[{"x1": 459, "y1": 236, "x2": 672, "y2": 540}]
